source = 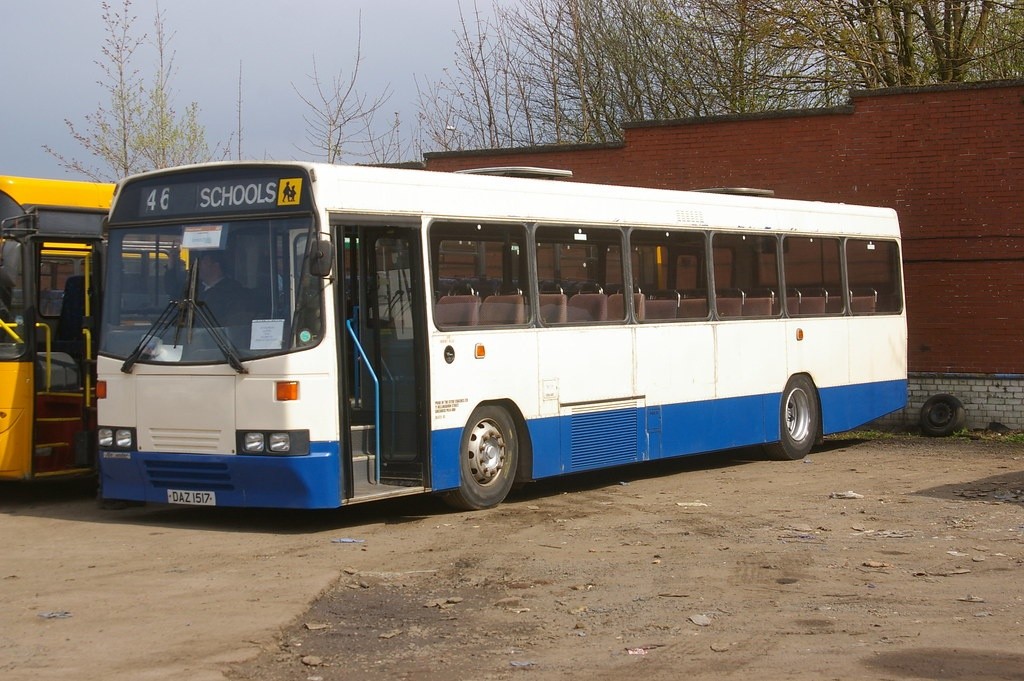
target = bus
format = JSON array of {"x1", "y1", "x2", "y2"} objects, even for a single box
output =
[
  {"x1": 0, "y1": 173, "x2": 194, "y2": 480},
  {"x1": 92, "y1": 158, "x2": 909, "y2": 514}
]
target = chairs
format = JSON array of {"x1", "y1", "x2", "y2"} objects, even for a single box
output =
[
  {"x1": 433, "y1": 277, "x2": 892, "y2": 329},
  {"x1": 37, "y1": 275, "x2": 90, "y2": 354}
]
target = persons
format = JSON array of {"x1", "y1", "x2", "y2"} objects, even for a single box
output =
[{"x1": 162, "y1": 248, "x2": 252, "y2": 328}]
